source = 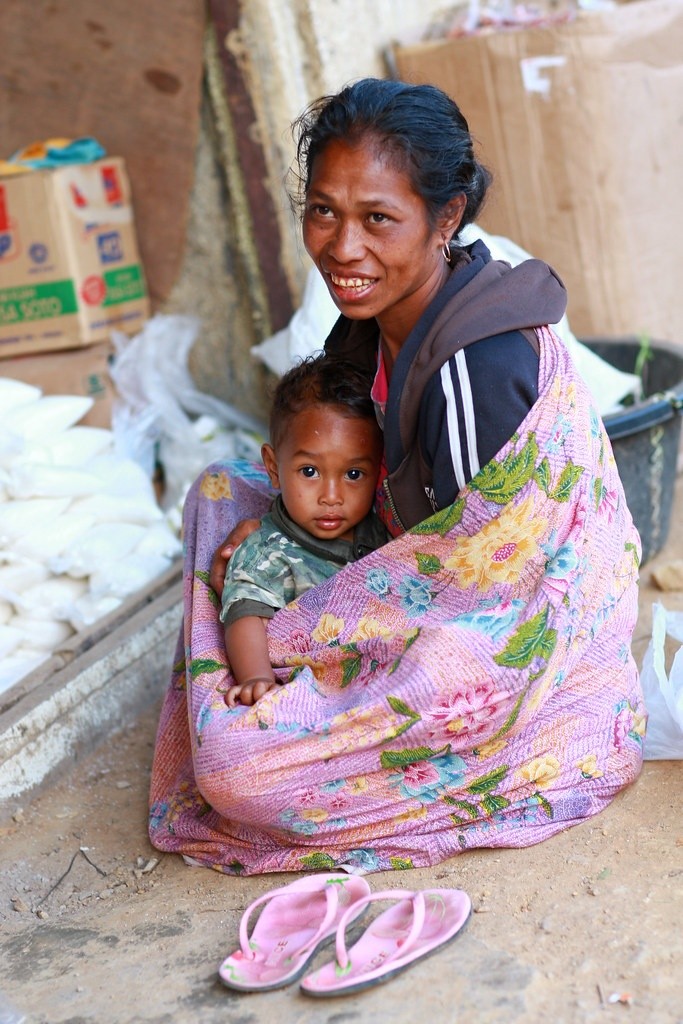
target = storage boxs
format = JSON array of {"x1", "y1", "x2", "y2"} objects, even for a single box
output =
[
  {"x1": 0, "y1": 335, "x2": 162, "y2": 482},
  {"x1": 0, "y1": 155, "x2": 150, "y2": 361},
  {"x1": 380, "y1": 1, "x2": 683, "y2": 346}
]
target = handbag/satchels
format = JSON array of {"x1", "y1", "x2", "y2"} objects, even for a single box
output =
[{"x1": 639, "y1": 600, "x2": 683, "y2": 761}]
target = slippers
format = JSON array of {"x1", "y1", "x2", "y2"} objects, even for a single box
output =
[
  {"x1": 218, "y1": 873, "x2": 370, "y2": 992},
  {"x1": 300, "y1": 888, "x2": 472, "y2": 996}
]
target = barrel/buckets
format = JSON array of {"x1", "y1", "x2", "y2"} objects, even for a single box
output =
[{"x1": 578, "y1": 335, "x2": 683, "y2": 566}]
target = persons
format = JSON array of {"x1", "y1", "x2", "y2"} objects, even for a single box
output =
[{"x1": 181, "y1": 80, "x2": 641, "y2": 847}]
